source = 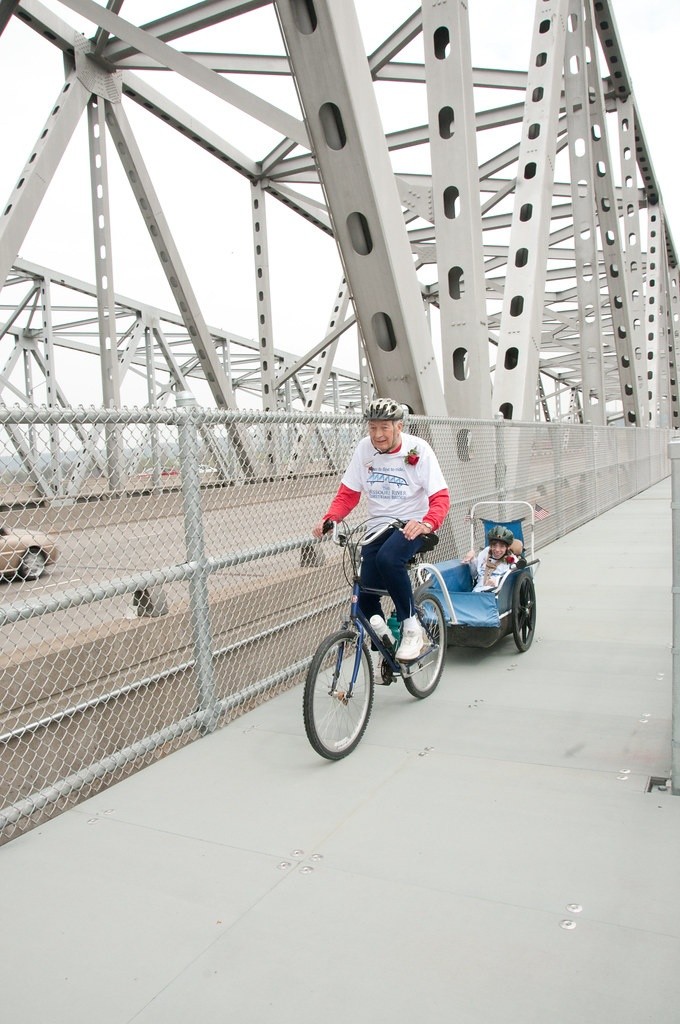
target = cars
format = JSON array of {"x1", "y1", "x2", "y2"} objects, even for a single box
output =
[
  {"x1": 138, "y1": 464, "x2": 217, "y2": 476},
  {"x1": 0, "y1": 523, "x2": 60, "y2": 581}
]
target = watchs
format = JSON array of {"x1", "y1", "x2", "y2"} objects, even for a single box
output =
[{"x1": 421, "y1": 522, "x2": 432, "y2": 532}]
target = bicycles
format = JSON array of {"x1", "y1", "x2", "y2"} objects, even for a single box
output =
[{"x1": 303, "y1": 515, "x2": 447, "y2": 761}]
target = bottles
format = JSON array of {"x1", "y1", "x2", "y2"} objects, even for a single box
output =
[
  {"x1": 387, "y1": 612, "x2": 400, "y2": 640},
  {"x1": 369, "y1": 615, "x2": 396, "y2": 646}
]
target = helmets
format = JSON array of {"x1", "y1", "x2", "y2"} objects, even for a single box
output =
[
  {"x1": 362, "y1": 399, "x2": 404, "y2": 421},
  {"x1": 487, "y1": 525, "x2": 514, "y2": 547}
]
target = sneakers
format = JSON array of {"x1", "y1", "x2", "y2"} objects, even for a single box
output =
[
  {"x1": 395, "y1": 628, "x2": 423, "y2": 660},
  {"x1": 374, "y1": 654, "x2": 385, "y2": 686}
]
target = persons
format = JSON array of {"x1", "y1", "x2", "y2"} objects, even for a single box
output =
[
  {"x1": 465, "y1": 526, "x2": 518, "y2": 603},
  {"x1": 312, "y1": 398, "x2": 450, "y2": 662}
]
